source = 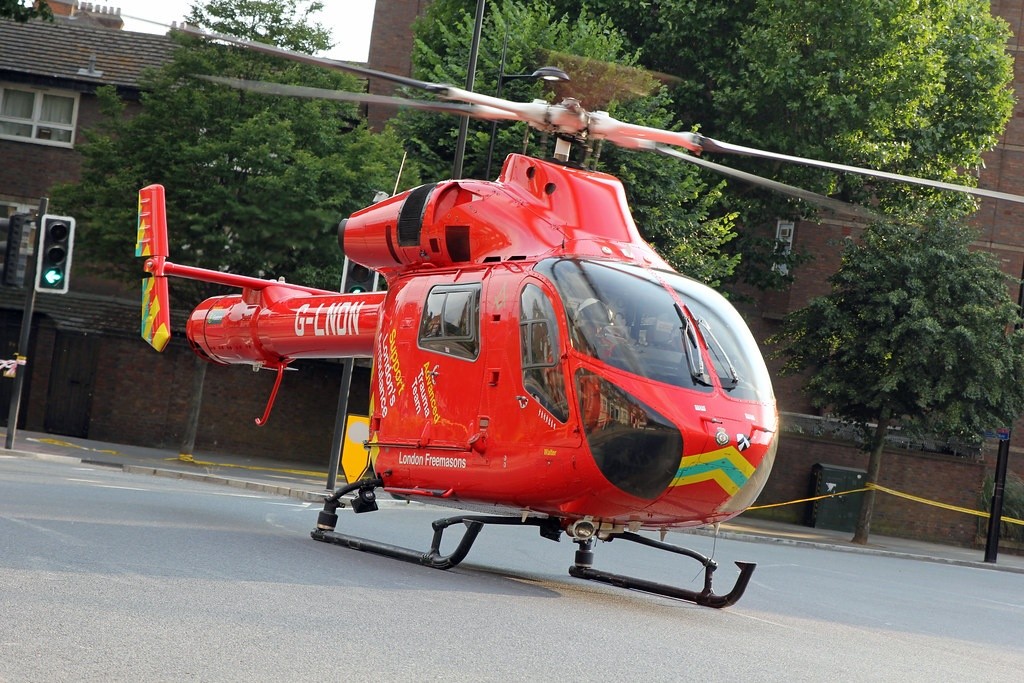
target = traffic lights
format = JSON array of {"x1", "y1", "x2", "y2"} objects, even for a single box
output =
[
  {"x1": 34, "y1": 214, "x2": 76, "y2": 295},
  {"x1": 0, "y1": 211, "x2": 27, "y2": 288}
]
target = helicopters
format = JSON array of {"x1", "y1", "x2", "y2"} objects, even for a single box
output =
[{"x1": 48, "y1": 0, "x2": 1023, "y2": 608}]
